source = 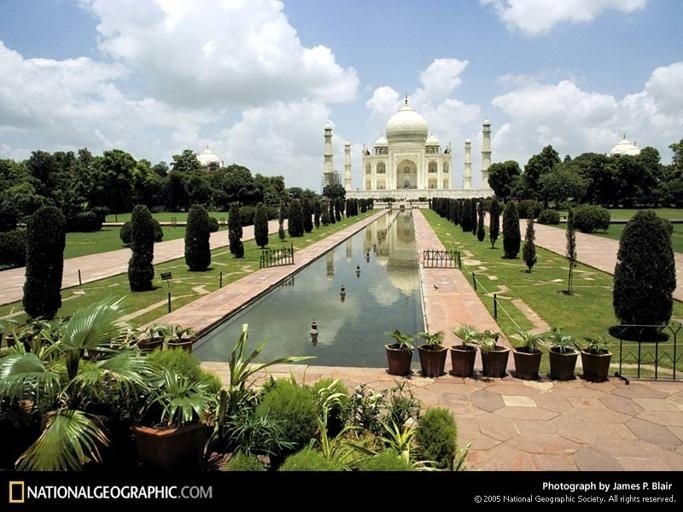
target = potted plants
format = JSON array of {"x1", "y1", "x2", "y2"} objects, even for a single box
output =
[
  {"x1": 512, "y1": 329, "x2": 543, "y2": 377},
  {"x1": 547, "y1": 327, "x2": 579, "y2": 378},
  {"x1": 449, "y1": 323, "x2": 483, "y2": 377},
  {"x1": 416, "y1": 330, "x2": 448, "y2": 376},
  {"x1": 477, "y1": 329, "x2": 511, "y2": 378},
  {"x1": 580, "y1": 330, "x2": 613, "y2": 382},
  {"x1": 383, "y1": 328, "x2": 414, "y2": 373}
]
